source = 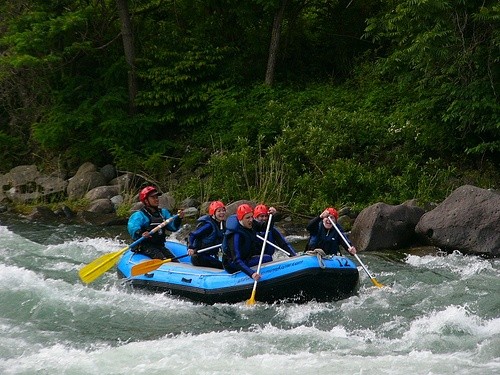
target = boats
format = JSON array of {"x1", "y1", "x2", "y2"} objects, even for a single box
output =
[{"x1": 115, "y1": 238, "x2": 362, "y2": 304}]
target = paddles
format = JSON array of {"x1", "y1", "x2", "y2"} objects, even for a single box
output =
[
  {"x1": 326, "y1": 215, "x2": 383, "y2": 287},
  {"x1": 246, "y1": 210, "x2": 274, "y2": 304},
  {"x1": 78, "y1": 210, "x2": 182, "y2": 284},
  {"x1": 255, "y1": 234, "x2": 293, "y2": 258},
  {"x1": 131, "y1": 243, "x2": 223, "y2": 277}
]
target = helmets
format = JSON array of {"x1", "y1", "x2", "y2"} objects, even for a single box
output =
[
  {"x1": 253, "y1": 205, "x2": 269, "y2": 218},
  {"x1": 209, "y1": 201, "x2": 226, "y2": 216},
  {"x1": 140, "y1": 186, "x2": 157, "y2": 202},
  {"x1": 236, "y1": 204, "x2": 253, "y2": 220},
  {"x1": 325, "y1": 208, "x2": 338, "y2": 221}
]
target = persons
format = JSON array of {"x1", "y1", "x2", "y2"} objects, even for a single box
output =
[
  {"x1": 127, "y1": 186, "x2": 185, "y2": 263},
  {"x1": 222, "y1": 204, "x2": 297, "y2": 281},
  {"x1": 187, "y1": 201, "x2": 226, "y2": 270},
  {"x1": 304, "y1": 207, "x2": 357, "y2": 256}
]
